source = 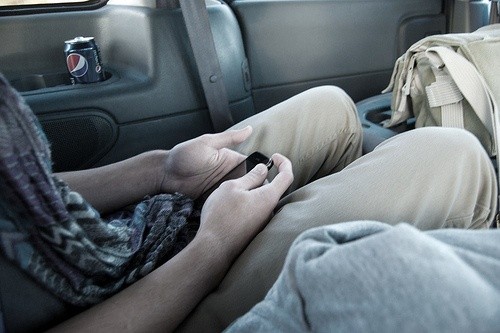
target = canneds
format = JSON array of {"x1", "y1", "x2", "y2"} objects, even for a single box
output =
[{"x1": 63, "y1": 35, "x2": 106, "y2": 84}]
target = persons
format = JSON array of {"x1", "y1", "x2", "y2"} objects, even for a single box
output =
[{"x1": 0, "y1": 69, "x2": 500, "y2": 333}]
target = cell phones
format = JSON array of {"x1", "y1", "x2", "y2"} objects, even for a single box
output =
[{"x1": 194, "y1": 151, "x2": 274, "y2": 213}]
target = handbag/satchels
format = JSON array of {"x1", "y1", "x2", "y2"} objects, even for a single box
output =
[{"x1": 381, "y1": 23, "x2": 499, "y2": 157}]
